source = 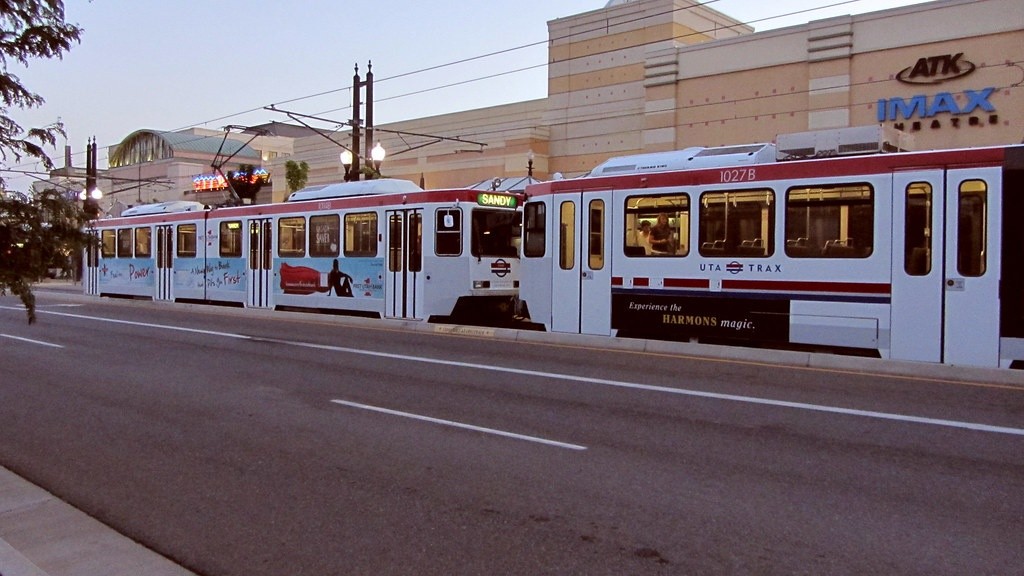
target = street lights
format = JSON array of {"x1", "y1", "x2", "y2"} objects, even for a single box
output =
[
  {"x1": 341, "y1": 60, "x2": 386, "y2": 182},
  {"x1": 79, "y1": 135, "x2": 103, "y2": 222}
]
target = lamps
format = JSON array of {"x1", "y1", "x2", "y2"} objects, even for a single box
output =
[
  {"x1": 340, "y1": 147, "x2": 353, "y2": 182},
  {"x1": 371, "y1": 141, "x2": 386, "y2": 176}
]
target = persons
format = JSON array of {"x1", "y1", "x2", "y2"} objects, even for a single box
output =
[{"x1": 637, "y1": 212, "x2": 674, "y2": 255}]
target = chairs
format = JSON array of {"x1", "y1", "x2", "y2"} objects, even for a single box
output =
[{"x1": 699, "y1": 237, "x2": 856, "y2": 257}]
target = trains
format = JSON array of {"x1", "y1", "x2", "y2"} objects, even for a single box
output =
[{"x1": 82, "y1": 124, "x2": 1023, "y2": 369}]
